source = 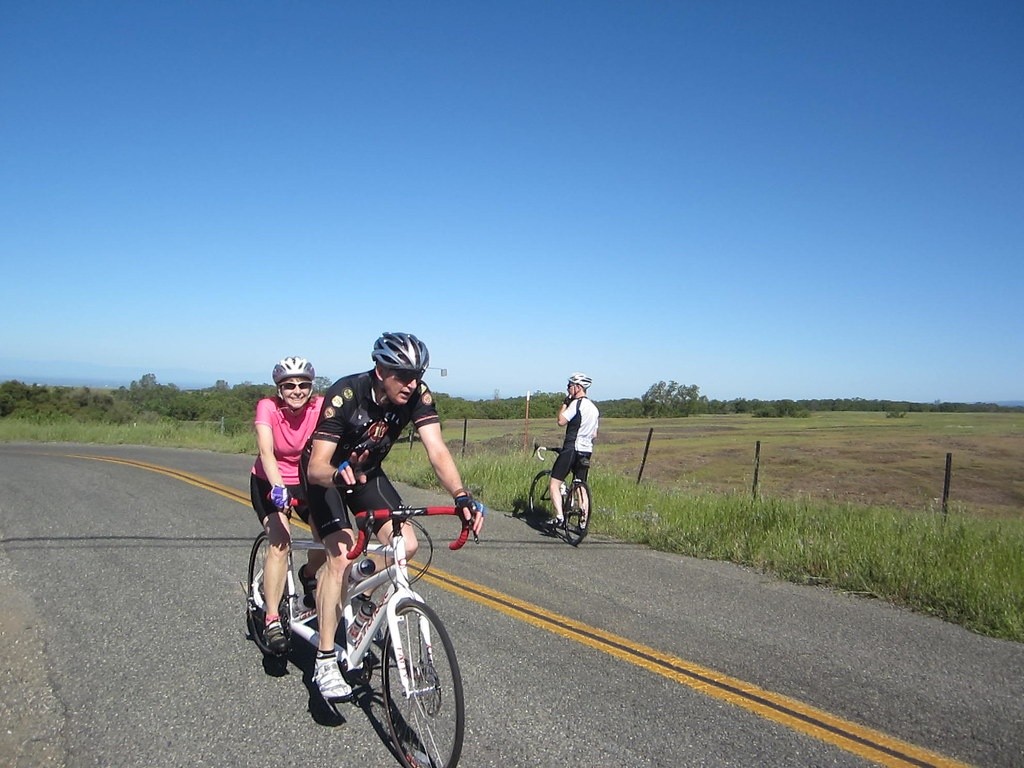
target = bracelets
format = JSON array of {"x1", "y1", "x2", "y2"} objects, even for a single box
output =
[{"x1": 332, "y1": 470, "x2": 337, "y2": 485}]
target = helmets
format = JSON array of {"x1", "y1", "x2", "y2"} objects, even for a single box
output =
[
  {"x1": 568, "y1": 373, "x2": 592, "y2": 389},
  {"x1": 272, "y1": 356, "x2": 316, "y2": 384},
  {"x1": 371, "y1": 331, "x2": 429, "y2": 373}
]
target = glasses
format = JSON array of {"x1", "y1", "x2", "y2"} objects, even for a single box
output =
[
  {"x1": 390, "y1": 367, "x2": 424, "y2": 384},
  {"x1": 568, "y1": 385, "x2": 574, "y2": 389},
  {"x1": 280, "y1": 381, "x2": 313, "y2": 389}
]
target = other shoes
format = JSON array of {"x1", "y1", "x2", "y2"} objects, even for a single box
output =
[
  {"x1": 574, "y1": 525, "x2": 587, "y2": 536},
  {"x1": 545, "y1": 516, "x2": 566, "y2": 527}
]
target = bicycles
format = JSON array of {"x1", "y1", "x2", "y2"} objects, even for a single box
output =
[
  {"x1": 529, "y1": 442, "x2": 591, "y2": 545},
  {"x1": 244, "y1": 496, "x2": 479, "y2": 768}
]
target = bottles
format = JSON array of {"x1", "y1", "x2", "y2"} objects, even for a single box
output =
[
  {"x1": 349, "y1": 558, "x2": 375, "y2": 584},
  {"x1": 560, "y1": 481, "x2": 567, "y2": 495},
  {"x1": 349, "y1": 601, "x2": 376, "y2": 639}
]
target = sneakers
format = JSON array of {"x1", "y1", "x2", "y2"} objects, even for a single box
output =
[
  {"x1": 298, "y1": 564, "x2": 318, "y2": 597},
  {"x1": 262, "y1": 618, "x2": 288, "y2": 653},
  {"x1": 312, "y1": 656, "x2": 353, "y2": 702}
]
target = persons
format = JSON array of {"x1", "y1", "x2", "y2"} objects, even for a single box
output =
[
  {"x1": 546, "y1": 374, "x2": 600, "y2": 530},
  {"x1": 249, "y1": 356, "x2": 325, "y2": 656},
  {"x1": 299, "y1": 331, "x2": 486, "y2": 703}
]
